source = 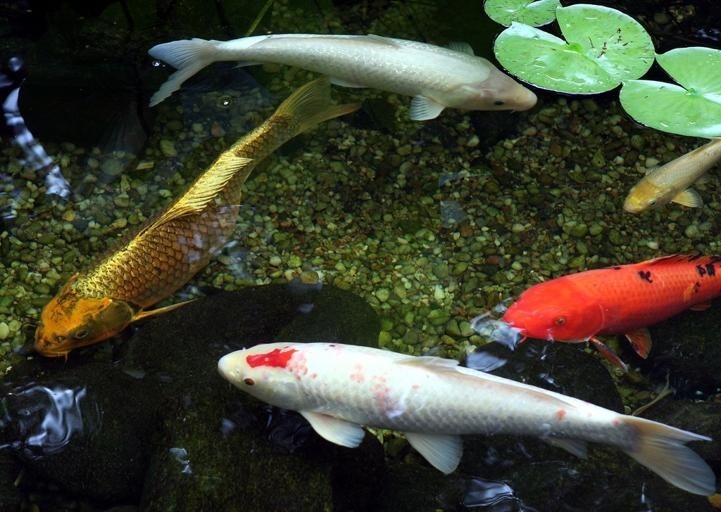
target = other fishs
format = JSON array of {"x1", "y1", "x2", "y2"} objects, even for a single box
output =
[
  {"x1": 618, "y1": 125, "x2": 720, "y2": 215},
  {"x1": 476, "y1": 253, "x2": 721, "y2": 372},
  {"x1": 1, "y1": 86, "x2": 73, "y2": 199},
  {"x1": 213, "y1": 340, "x2": 721, "y2": 499},
  {"x1": 148, "y1": 31, "x2": 539, "y2": 125},
  {"x1": 32, "y1": 74, "x2": 362, "y2": 363}
]
